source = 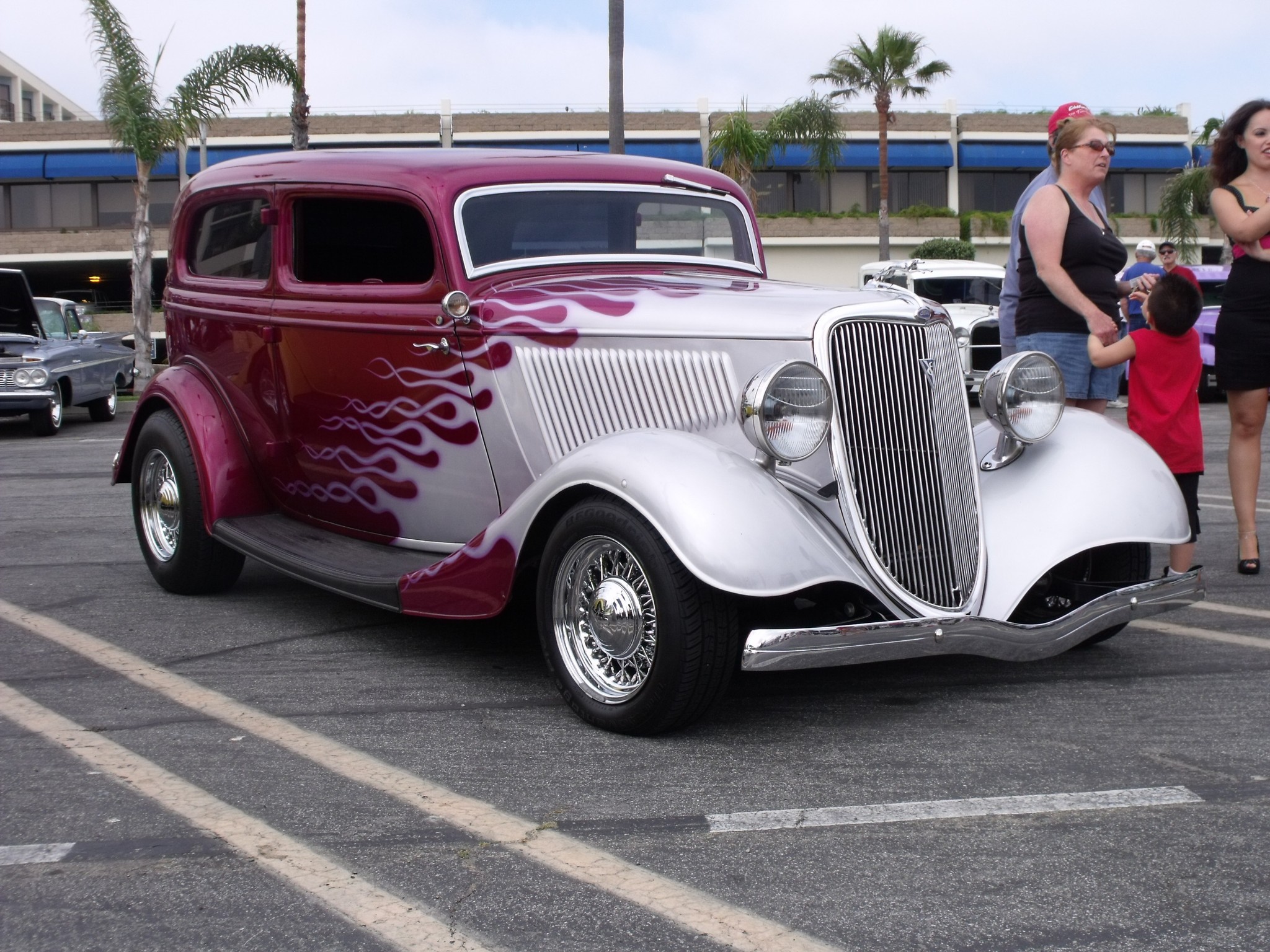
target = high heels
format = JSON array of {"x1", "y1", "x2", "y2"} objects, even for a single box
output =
[{"x1": 1237, "y1": 533, "x2": 1259, "y2": 575}]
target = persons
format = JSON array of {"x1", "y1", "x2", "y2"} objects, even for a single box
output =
[
  {"x1": 1087, "y1": 274, "x2": 1207, "y2": 577},
  {"x1": 997, "y1": 103, "x2": 1202, "y2": 415},
  {"x1": 1207, "y1": 100, "x2": 1270, "y2": 576}
]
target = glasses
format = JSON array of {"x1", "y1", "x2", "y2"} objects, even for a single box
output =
[
  {"x1": 1160, "y1": 250, "x2": 1176, "y2": 255},
  {"x1": 1067, "y1": 140, "x2": 1116, "y2": 157}
]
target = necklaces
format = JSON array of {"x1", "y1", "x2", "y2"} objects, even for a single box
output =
[
  {"x1": 1079, "y1": 202, "x2": 1105, "y2": 235},
  {"x1": 1247, "y1": 177, "x2": 1270, "y2": 195}
]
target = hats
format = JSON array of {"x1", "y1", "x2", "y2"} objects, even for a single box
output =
[
  {"x1": 1048, "y1": 102, "x2": 1096, "y2": 135},
  {"x1": 1159, "y1": 242, "x2": 1175, "y2": 250},
  {"x1": 1136, "y1": 240, "x2": 1156, "y2": 252}
]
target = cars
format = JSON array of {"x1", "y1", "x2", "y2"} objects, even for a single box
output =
[
  {"x1": 109, "y1": 141, "x2": 1213, "y2": 734},
  {"x1": 1170, "y1": 264, "x2": 1230, "y2": 370},
  {"x1": 0, "y1": 266, "x2": 137, "y2": 430},
  {"x1": 858, "y1": 257, "x2": 1015, "y2": 392}
]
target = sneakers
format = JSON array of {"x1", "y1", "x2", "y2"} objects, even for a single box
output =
[{"x1": 1107, "y1": 399, "x2": 1128, "y2": 408}]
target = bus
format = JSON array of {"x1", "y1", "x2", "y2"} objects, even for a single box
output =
[{"x1": 0, "y1": 249, "x2": 170, "y2": 338}]
